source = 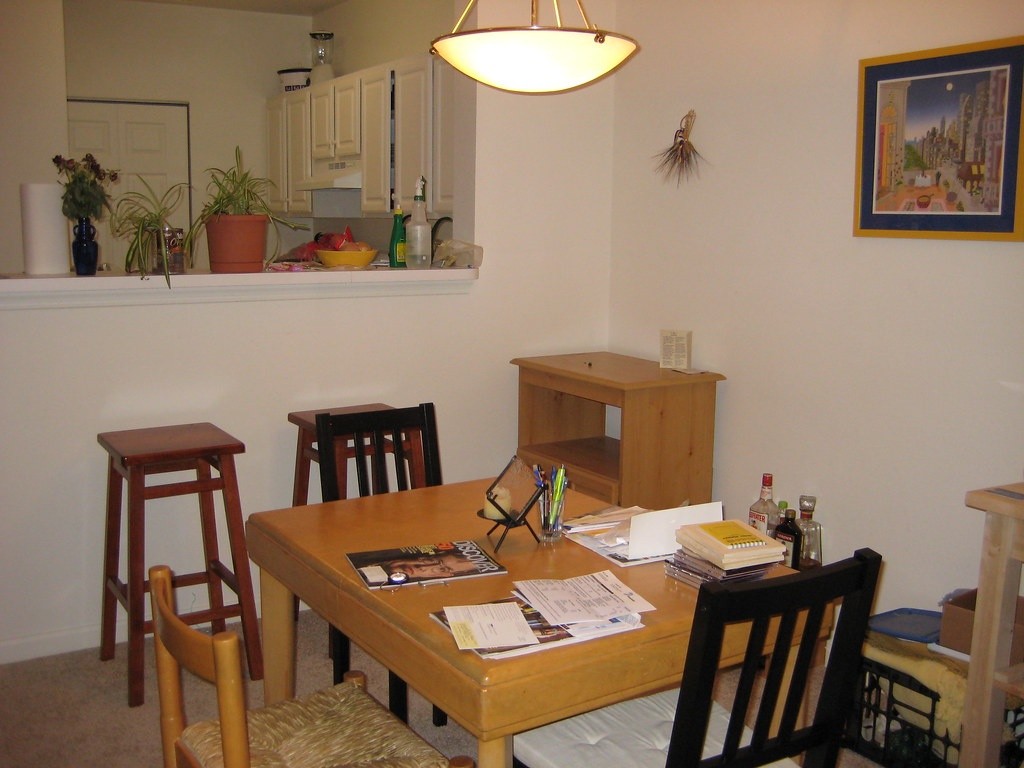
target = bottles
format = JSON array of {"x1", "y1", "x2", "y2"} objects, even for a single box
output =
[
  {"x1": 748, "y1": 473, "x2": 781, "y2": 540},
  {"x1": 774, "y1": 509, "x2": 801, "y2": 572},
  {"x1": 777, "y1": 500, "x2": 788, "y2": 517},
  {"x1": 795, "y1": 495, "x2": 822, "y2": 571}
]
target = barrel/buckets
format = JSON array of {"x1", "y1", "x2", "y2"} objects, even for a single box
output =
[{"x1": 277, "y1": 68, "x2": 310, "y2": 95}]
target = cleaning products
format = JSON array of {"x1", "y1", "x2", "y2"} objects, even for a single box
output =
[{"x1": 389, "y1": 175, "x2": 432, "y2": 270}]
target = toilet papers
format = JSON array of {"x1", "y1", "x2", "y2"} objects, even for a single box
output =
[{"x1": 20, "y1": 182, "x2": 71, "y2": 275}]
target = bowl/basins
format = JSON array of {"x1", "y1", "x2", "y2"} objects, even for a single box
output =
[{"x1": 315, "y1": 248, "x2": 379, "y2": 268}]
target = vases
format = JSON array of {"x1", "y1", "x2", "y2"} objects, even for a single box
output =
[{"x1": 73, "y1": 217, "x2": 99, "y2": 276}]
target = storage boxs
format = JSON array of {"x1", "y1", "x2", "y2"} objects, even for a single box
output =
[{"x1": 845, "y1": 588, "x2": 1024, "y2": 768}]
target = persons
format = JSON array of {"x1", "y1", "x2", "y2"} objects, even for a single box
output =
[{"x1": 359, "y1": 552, "x2": 479, "y2": 582}]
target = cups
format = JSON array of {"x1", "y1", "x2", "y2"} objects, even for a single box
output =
[{"x1": 538, "y1": 500, "x2": 564, "y2": 541}]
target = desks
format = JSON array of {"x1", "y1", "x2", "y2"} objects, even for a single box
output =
[
  {"x1": 957, "y1": 480, "x2": 1024, "y2": 767},
  {"x1": 243, "y1": 476, "x2": 834, "y2": 768}
]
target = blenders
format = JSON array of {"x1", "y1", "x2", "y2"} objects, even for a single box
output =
[{"x1": 309, "y1": 30, "x2": 336, "y2": 87}]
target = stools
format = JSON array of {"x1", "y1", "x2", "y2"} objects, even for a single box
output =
[
  {"x1": 287, "y1": 404, "x2": 423, "y2": 506},
  {"x1": 98, "y1": 423, "x2": 263, "y2": 706}
]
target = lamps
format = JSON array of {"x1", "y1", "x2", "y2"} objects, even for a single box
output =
[{"x1": 430, "y1": 0, "x2": 637, "y2": 93}]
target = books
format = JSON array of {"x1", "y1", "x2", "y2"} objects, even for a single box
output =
[
  {"x1": 659, "y1": 330, "x2": 690, "y2": 369},
  {"x1": 346, "y1": 539, "x2": 508, "y2": 591},
  {"x1": 664, "y1": 520, "x2": 786, "y2": 588},
  {"x1": 428, "y1": 594, "x2": 571, "y2": 659}
]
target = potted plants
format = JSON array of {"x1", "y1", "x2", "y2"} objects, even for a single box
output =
[
  {"x1": 109, "y1": 173, "x2": 192, "y2": 291},
  {"x1": 182, "y1": 146, "x2": 309, "y2": 274}
]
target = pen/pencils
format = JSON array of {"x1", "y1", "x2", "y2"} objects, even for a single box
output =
[{"x1": 532, "y1": 463, "x2": 569, "y2": 532}]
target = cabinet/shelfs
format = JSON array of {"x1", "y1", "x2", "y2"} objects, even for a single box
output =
[
  {"x1": 511, "y1": 352, "x2": 726, "y2": 509},
  {"x1": 266, "y1": 50, "x2": 453, "y2": 221}
]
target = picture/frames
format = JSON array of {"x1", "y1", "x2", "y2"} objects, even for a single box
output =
[{"x1": 854, "y1": 34, "x2": 1024, "y2": 241}]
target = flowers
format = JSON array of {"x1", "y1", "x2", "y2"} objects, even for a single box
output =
[{"x1": 52, "y1": 152, "x2": 119, "y2": 222}]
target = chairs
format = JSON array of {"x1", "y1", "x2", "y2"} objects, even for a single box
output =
[
  {"x1": 511, "y1": 547, "x2": 882, "y2": 768},
  {"x1": 149, "y1": 565, "x2": 475, "y2": 768},
  {"x1": 315, "y1": 402, "x2": 447, "y2": 731}
]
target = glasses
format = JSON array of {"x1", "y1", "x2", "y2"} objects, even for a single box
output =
[{"x1": 423, "y1": 555, "x2": 456, "y2": 574}]
